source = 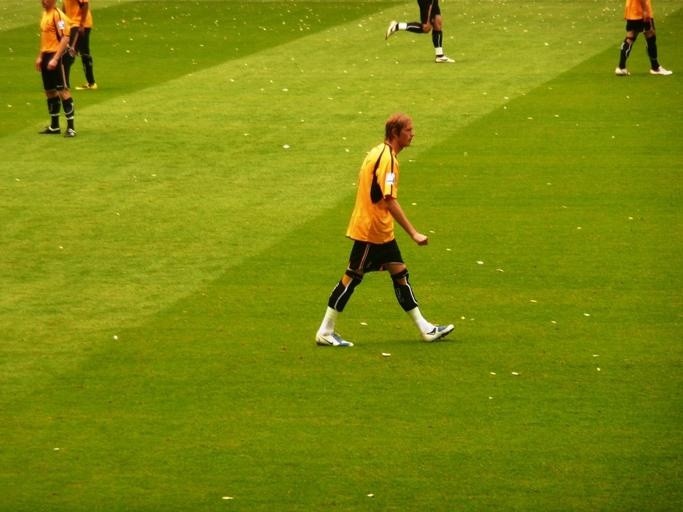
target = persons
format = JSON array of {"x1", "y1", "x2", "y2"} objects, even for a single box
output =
[
  {"x1": 34, "y1": 0, "x2": 77, "y2": 139},
  {"x1": 615, "y1": 0, "x2": 673, "y2": 76},
  {"x1": 58, "y1": 0, "x2": 98, "y2": 92},
  {"x1": 314, "y1": 111, "x2": 455, "y2": 347},
  {"x1": 384, "y1": 0, "x2": 456, "y2": 64}
]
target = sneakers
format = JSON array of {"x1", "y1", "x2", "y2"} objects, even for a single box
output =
[
  {"x1": 435, "y1": 55, "x2": 453, "y2": 63},
  {"x1": 39, "y1": 126, "x2": 61, "y2": 134},
  {"x1": 421, "y1": 324, "x2": 455, "y2": 341},
  {"x1": 616, "y1": 67, "x2": 630, "y2": 75},
  {"x1": 386, "y1": 21, "x2": 397, "y2": 38},
  {"x1": 80, "y1": 83, "x2": 96, "y2": 89},
  {"x1": 649, "y1": 65, "x2": 672, "y2": 75},
  {"x1": 315, "y1": 330, "x2": 353, "y2": 346},
  {"x1": 64, "y1": 127, "x2": 75, "y2": 136}
]
